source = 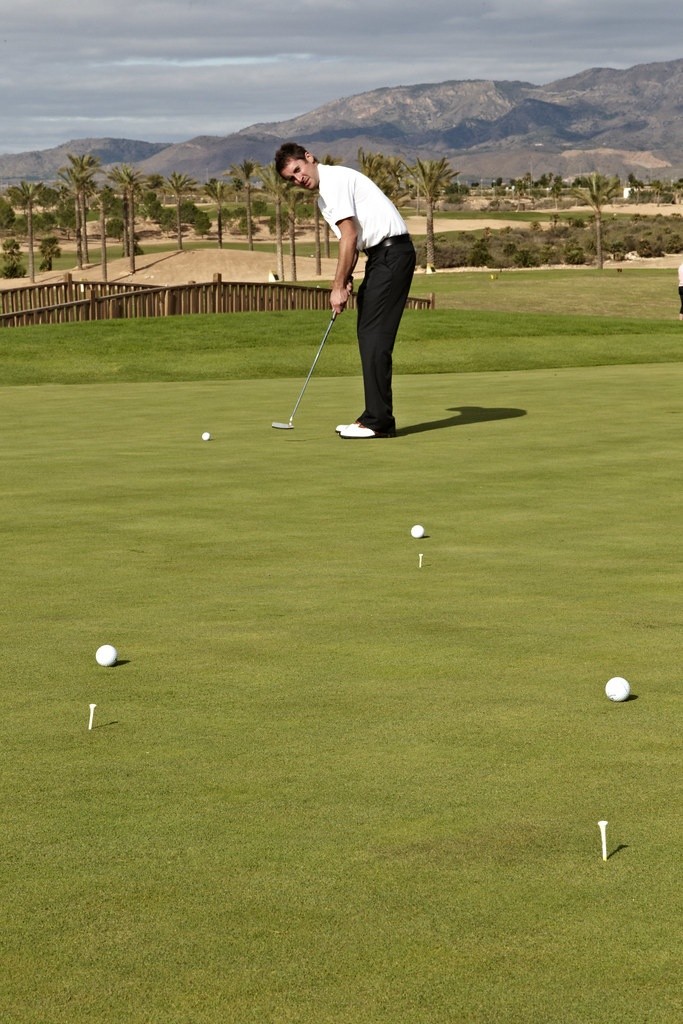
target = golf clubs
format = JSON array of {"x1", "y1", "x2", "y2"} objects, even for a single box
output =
[{"x1": 271, "y1": 276, "x2": 354, "y2": 429}]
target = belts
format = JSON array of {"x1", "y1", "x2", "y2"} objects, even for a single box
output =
[{"x1": 364, "y1": 235, "x2": 412, "y2": 256}]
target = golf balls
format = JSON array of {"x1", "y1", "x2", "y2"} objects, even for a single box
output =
[
  {"x1": 410, "y1": 524, "x2": 425, "y2": 539},
  {"x1": 604, "y1": 677, "x2": 631, "y2": 702},
  {"x1": 201, "y1": 431, "x2": 211, "y2": 442},
  {"x1": 95, "y1": 644, "x2": 118, "y2": 667}
]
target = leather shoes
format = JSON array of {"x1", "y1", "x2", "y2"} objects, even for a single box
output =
[
  {"x1": 339, "y1": 423, "x2": 396, "y2": 438},
  {"x1": 335, "y1": 420, "x2": 360, "y2": 433}
]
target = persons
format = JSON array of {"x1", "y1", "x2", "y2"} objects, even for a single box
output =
[
  {"x1": 677, "y1": 262, "x2": 683, "y2": 321},
  {"x1": 274, "y1": 144, "x2": 416, "y2": 438}
]
